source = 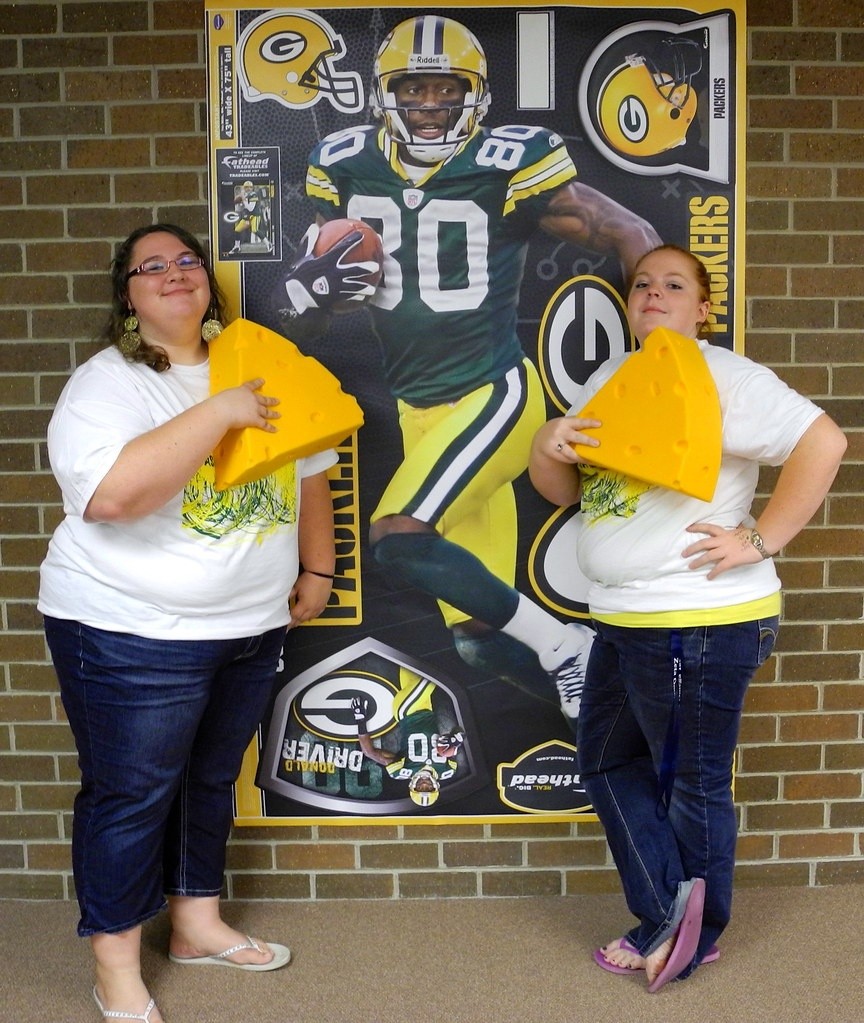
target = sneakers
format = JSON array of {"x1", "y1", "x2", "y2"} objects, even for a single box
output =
[
  {"x1": 228, "y1": 246, "x2": 242, "y2": 255},
  {"x1": 266, "y1": 242, "x2": 273, "y2": 253},
  {"x1": 538, "y1": 622, "x2": 597, "y2": 720}
]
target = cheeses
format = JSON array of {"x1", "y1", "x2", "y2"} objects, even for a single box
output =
[
  {"x1": 209, "y1": 318, "x2": 365, "y2": 492},
  {"x1": 567, "y1": 328, "x2": 724, "y2": 503}
]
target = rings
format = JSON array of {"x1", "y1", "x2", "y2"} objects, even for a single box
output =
[{"x1": 556, "y1": 443, "x2": 566, "y2": 451}]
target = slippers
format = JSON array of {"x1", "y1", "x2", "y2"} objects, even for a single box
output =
[
  {"x1": 91, "y1": 984, "x2": 156, "y2": 1023},
  {"x1": 645, "y1": 877, "x2": 707, "y2": 996},
  {"x1": 167, "y1": 933, "x2": 292, "y2": 972},
  {"x1": 592, "y1": 934, "x2": 721, "y2": 975}
]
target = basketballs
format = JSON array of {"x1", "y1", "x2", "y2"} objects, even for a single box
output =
[{"x1": 311, "y1": 217, "x2": 383, "y2": 311}]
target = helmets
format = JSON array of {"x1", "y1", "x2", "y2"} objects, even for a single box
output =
[
  {"x1": 409, "y1": 770, "x2": 439, "y2": 807},
  {"x1": 373, "y1": 15, "x2": 489, "y2": 164},
  {"x1": 594, "y1": 52, "x2": 701, "y2": 160},
  {"x1": 243, "y1": 181, "x2": 253, "y2": 190}
]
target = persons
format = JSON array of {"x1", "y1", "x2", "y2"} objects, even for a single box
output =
[
  {"x1": 228, "y1": 180, "x2": 272, "y2": 254},
  {"x1": 35, "y1": 224, "x2": 339, "y2": 1023},
  {"x1": 350, "y1": 667, "x2": 464, "y2": 806},
  {"x1": 268, "y1": 17, "x2": 664, "y2": 725},
  {"x1": 530, "y1": 246, "x2": 847, "y2": 993}
]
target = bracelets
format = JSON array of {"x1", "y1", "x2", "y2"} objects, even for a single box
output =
[
  {"x1": 308, "y1": 571, "x2": 335, "y2": 579},
  {"x1": 751, "y1": 529, "x2": 772, "y2": 559}
]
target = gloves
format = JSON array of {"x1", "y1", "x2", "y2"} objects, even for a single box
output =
[
  {"x1": 283, "y1": 222, "x2": 380, "y2": 330},
  {"x1": 437, "y1": 731, "x2": 464, "y2": 755},
  {"x1": 350, "y1": 696, "x2": 369, "y2": 720}
]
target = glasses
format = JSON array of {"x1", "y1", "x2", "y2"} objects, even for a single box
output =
[{"x1": 127, "y1": 254, "x2": 205, "y2": 280}]
